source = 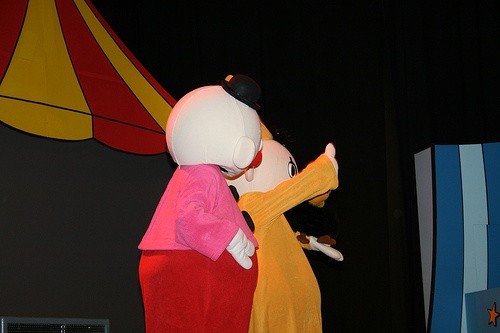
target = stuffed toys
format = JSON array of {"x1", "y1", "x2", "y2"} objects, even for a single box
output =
[
  {"x1": 140, "y1": 73, "x2": 260, "y2": 333},
  {"x1": 227, "y1": 107, "x2": 344, "y2": 333}
]
